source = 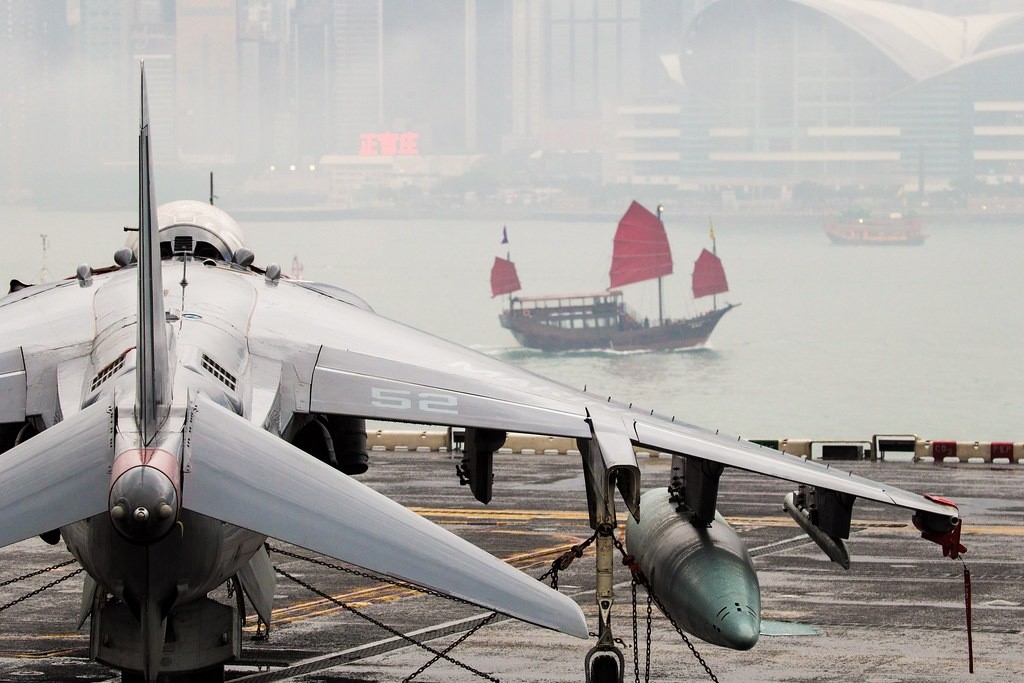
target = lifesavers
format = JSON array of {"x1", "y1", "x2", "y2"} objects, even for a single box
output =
[{"x1": 522, "y1": 309, "x2": 530, "y2": 318}]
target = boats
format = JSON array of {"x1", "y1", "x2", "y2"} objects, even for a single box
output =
[{"x1": 823, "y1": 216, "x2": 930, "y2": 246}]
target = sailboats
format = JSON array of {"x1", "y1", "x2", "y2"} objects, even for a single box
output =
[{"x1": 489, "y1": 200, "x2": 743, "y2": 352}]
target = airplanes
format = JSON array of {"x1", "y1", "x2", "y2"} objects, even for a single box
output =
[{"x1": 0, "y1": 57, "x2": 973, "y2": 683}]
